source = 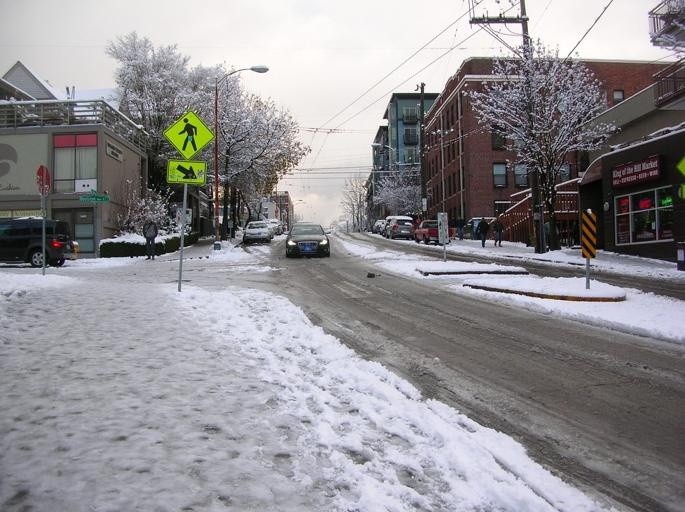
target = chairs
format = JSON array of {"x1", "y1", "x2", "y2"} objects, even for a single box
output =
[
  {"x1": 145, "y1": 255, "x2": 155, "y2": 260},
  {"x1": 494, "y1": 243, "x2": 502, "y2": 247}
]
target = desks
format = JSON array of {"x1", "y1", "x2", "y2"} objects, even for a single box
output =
[
  {"x1": 269, "y1": 172, "x2": 295, "y2": 198},
  {"x1": 369, "y1": 142, "x2": 397, "y2": 182},
  {"x1": 339, "y1": 188, "x2": 363, "y2": 229},
  {"x1": 432, "y1": 111, "x2": 456, "y2": 212},
  {"x1": 288, "y1": 199, "x2": 305, "y2": 227},
  {"x1": 213, "y1": 66, "x2": 269, "y2": 240}
]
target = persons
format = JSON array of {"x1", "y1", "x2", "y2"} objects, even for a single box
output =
[
  {"x1": 143, "y1": 216, "x2": 158, "y2": 260},
  {"x1": 475, "y1": 218, "x2": 505, "y2": 247},
  {"x1": 449, "y1": 215, "x2": 465, "y2": 240}
]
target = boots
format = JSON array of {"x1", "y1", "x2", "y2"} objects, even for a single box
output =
[{"x1": 0, "y1": 215, "x2": 71, "y2": 269}]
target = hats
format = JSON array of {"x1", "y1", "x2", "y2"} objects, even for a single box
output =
[
  {"x1": 463, "y1": 216, "x2": 497, "y2": 239},
  {"x1": 367, "y1": 215, "x2": 415, "y2": 241},
  {"x1": 285, "y1": 223, "x2": 332, "y2": 259},
  {"x1": 242, "y1": 218, "x2": 288, "y2": 244}
]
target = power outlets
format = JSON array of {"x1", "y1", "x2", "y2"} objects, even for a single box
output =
[{"x1": 414, "y1": 219, "x2": 454, "y2": 246}]
